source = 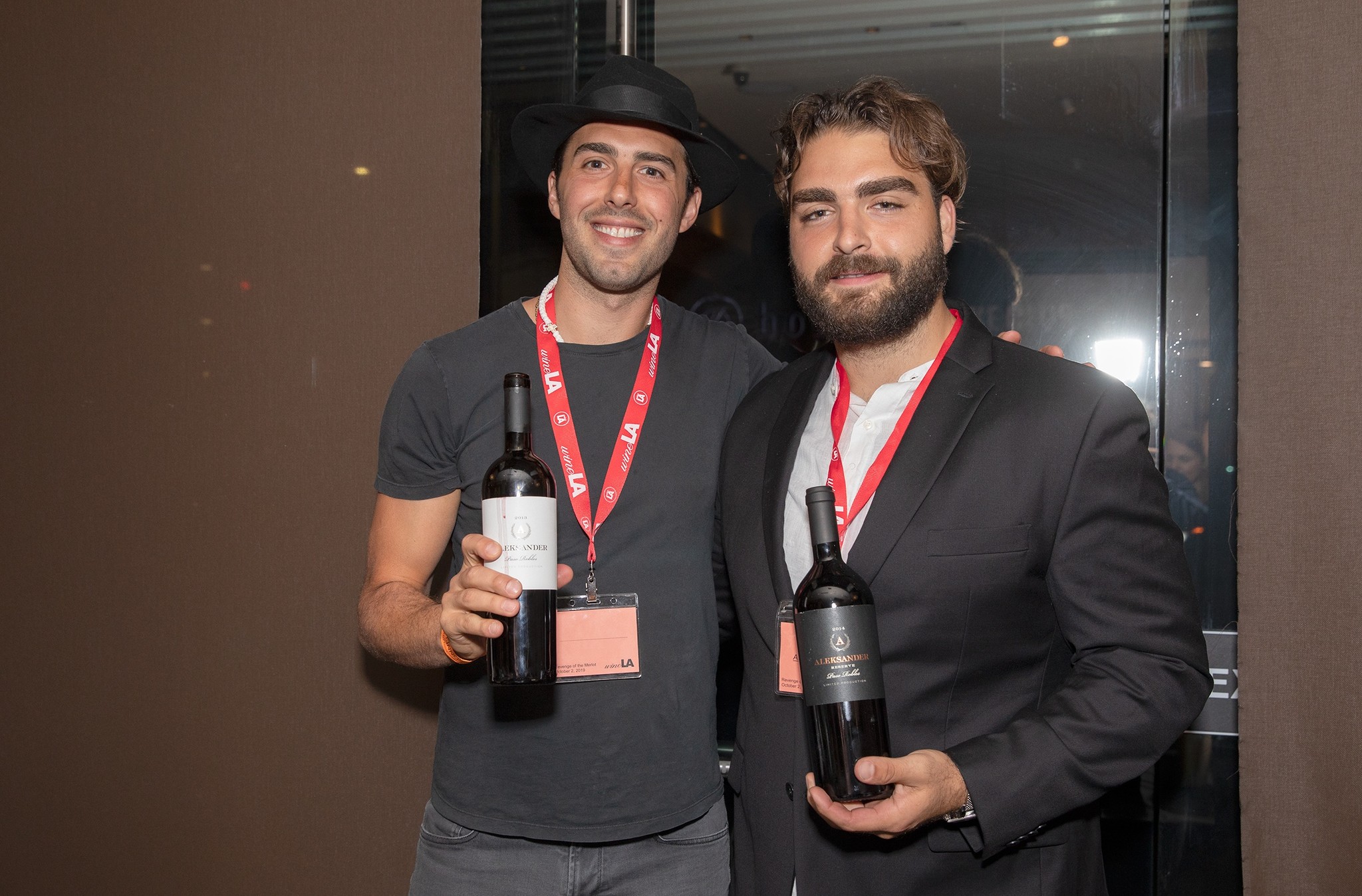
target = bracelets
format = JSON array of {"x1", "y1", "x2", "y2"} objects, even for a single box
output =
[{"x1": 441, "y1": 629, "x2": 475, "y2": 666}]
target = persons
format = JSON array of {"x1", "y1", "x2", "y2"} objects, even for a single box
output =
[
  {"x1": 950, "y1": 235, "x2": 1024, "y2": 337},
  {"x1": 355, "y1": 53, "x2": 1100, "y2": 896},
  {"x1": 715, "y1": 68, "x2": 1215, "y2": 896},
  {"x1": 1170, "y1": 431, "x2": 1210, "y2": 497}
]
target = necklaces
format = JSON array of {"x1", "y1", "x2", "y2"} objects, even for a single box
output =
[{"x1": 538, "y1": 274, "x2": 564, "y2": 344}]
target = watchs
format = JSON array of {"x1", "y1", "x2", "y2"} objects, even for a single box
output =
[{"x1": 942, "y1": 790, "x2": 977, "y2": 823}]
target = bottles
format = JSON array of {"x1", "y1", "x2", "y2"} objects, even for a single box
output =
[
  {"x1": 481, "y1": 371, "x2": 559, "y2": 686},
  {"x1": 792, "y1": 484, "x2": 896, "y2": 801}
]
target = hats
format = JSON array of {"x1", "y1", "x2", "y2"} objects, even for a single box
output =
[{"x1": 510, "y1": 54, "x2": 741, "y2": 216}]
